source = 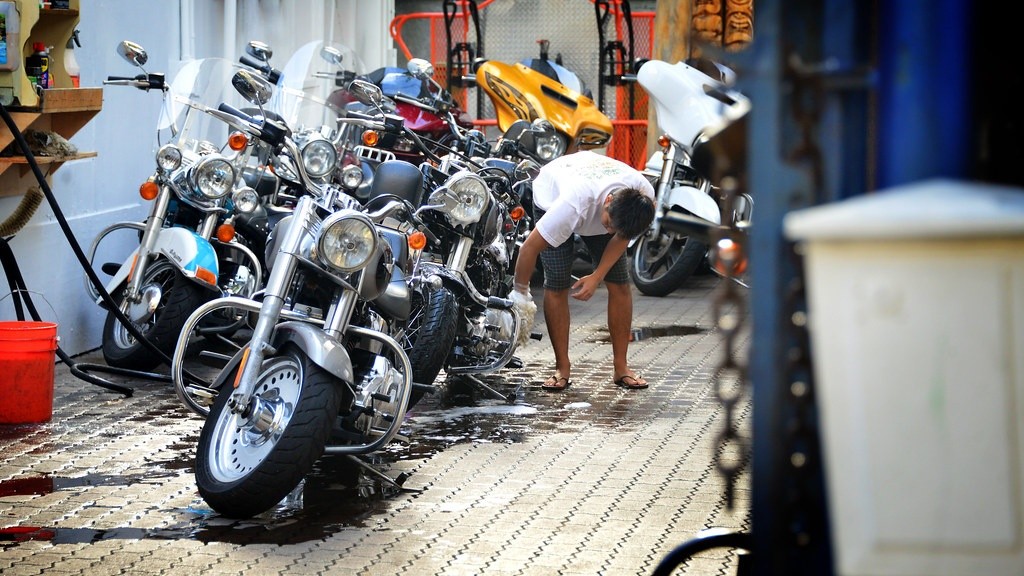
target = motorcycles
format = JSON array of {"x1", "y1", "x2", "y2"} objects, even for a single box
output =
[{"x1": 99, "y1": 34, "x2": 760, "y2": 525}]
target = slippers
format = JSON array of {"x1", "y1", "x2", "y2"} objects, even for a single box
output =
[
  {"x1": 614, "y1": 374, "x2": 649, "y2": 389},
  {"x1": 542, "y1": 376, "x2": 572, "y2": 389}
]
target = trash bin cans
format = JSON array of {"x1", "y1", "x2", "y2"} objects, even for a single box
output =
[{"x1": 782, "y1": 178, "x2": 1024, "y2": 576}]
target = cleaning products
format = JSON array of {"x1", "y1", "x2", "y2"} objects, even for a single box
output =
[
  {"x1": 25, "y1": 41, "x2": 56, "y2": 96},
  {"x1": 62, "y1": 28, "x2": 82, "y2": 88}
]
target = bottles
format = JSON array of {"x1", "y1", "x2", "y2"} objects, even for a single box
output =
[{"x1": 25, "y1": 41, "x2": 56, "y2": 90}]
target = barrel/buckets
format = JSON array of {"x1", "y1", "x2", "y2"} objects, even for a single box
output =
[{"x1": 0, "y1": 290, "x2": 58, "y2": 423}]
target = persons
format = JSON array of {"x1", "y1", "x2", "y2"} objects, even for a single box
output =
[{"x1": 510, "y1": 146, "x2": 656, "y2": 390}]
